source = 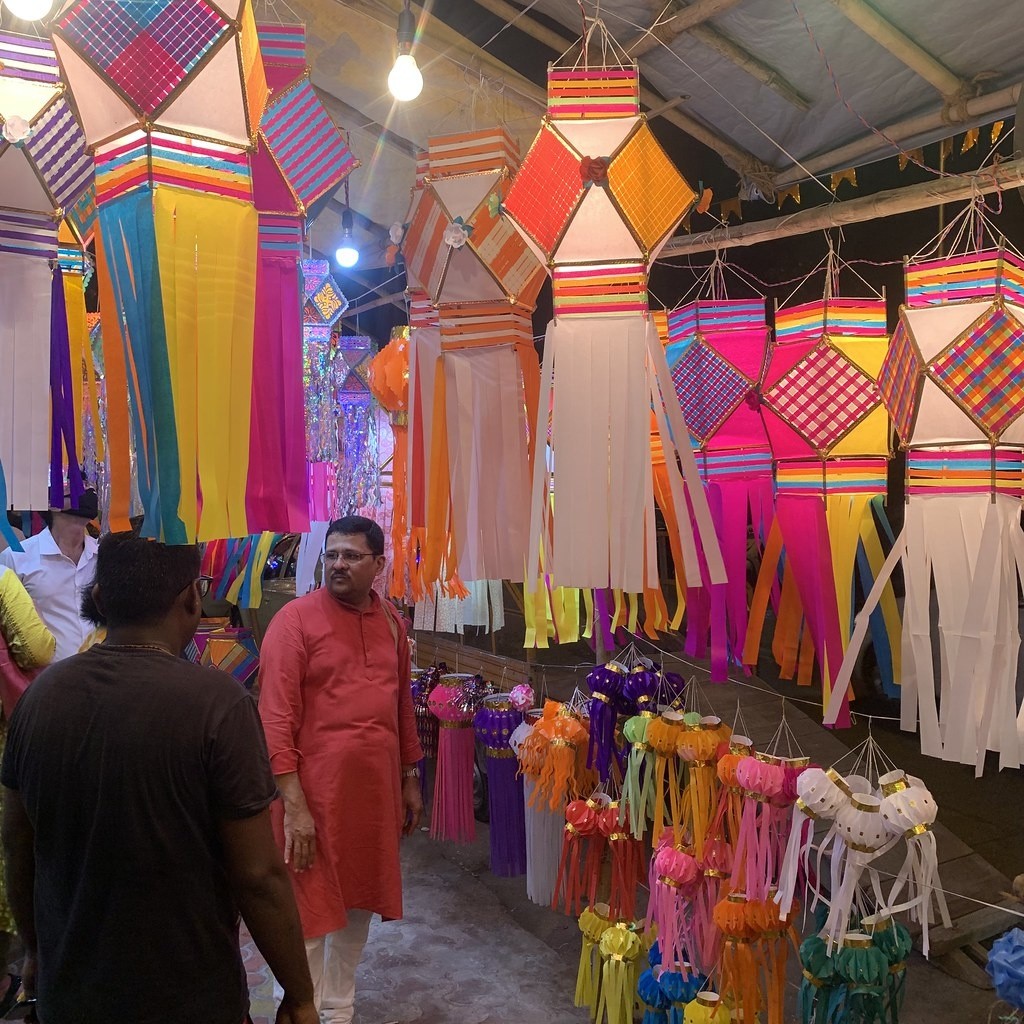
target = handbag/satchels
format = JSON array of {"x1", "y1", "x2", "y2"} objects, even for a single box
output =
[{"x1": 0, "y1": 631, "x2": 54, "y2": 724}]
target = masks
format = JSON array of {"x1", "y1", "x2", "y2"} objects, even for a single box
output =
[{"x1": 56, "y1": 488, "x2": 99, "y2": 520}]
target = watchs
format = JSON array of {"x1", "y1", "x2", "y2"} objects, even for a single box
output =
[{"x1": 402, "y1": 767, "x2": 421, "y2": 779}]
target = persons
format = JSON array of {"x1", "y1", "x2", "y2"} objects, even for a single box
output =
[
  {"x1": 0, "y1": 469, "x2": 321, "y2": 1024},
  {"x1": 258, "y1": 516, "x2": 424, "y2": 1024}
]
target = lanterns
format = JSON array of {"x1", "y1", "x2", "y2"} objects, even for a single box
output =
[
  {"x1": 0, "y1": 0, "x2": 1024, "y2": 779},
  {"x1": 184, "y1": 617, "x2": 952, "y2": 1024}
]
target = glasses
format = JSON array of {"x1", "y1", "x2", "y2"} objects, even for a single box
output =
[
  {"x1": 177, "y1": 573, "x2": 214, "y2": 598},
  {"x1": 318, "y1": 550, "x2": 375, "y2": 562}
]
